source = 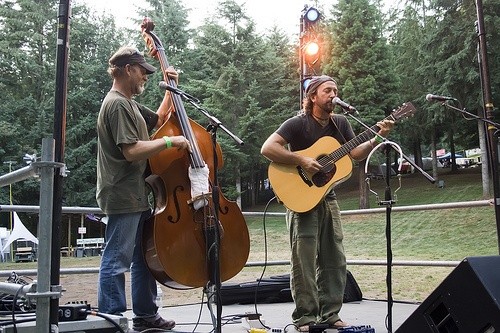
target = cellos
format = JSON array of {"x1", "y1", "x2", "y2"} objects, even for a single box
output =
[{"x1": 140, "y1": 17, "x2": 250, "y2": 333}]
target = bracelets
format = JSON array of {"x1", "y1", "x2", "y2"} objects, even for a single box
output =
[
  {"x1": 375, "y1": 135, "x2": 384, "y2": 143},
  {"x1": 163, "y1": 135, "x2": 172, "y2": 149}
]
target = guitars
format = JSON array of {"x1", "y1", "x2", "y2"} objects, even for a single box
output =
[{"x1": 268, "y1": 102, "x2": 418, "y2": 214}]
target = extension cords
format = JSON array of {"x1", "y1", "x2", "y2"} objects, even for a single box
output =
[{"x1": 240, "y1": 316, "x2": 264, "y2": 329}]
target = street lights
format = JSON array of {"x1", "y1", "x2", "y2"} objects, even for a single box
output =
[{"x1": 3, "y1": 161, "x2": 18, "y2": 261}]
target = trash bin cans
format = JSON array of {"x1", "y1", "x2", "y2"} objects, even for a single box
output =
[
  {"x1": 74, "y1": 248, "x2": 77, "y2": 257},
  {"x1": 92, "y1": 248, "x2": 101, "y2": 256},
  {"x1": 77, "y1": 247, "x2": 84, "y2": 257},
  {"x1": 85, "y1": 249, "x2": 92, "y2": 257}
]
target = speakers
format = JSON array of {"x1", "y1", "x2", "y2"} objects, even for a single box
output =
[{"x1": 394, "y1": 254, "x2": 500, "y2": 333}]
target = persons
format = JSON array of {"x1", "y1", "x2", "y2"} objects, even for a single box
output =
[
  {"x1": 261, "y1": 75, "x2": 394, "y2": 332},
  {"x1": 96, "y1": 47, "x2": 194, "y2": 331}
]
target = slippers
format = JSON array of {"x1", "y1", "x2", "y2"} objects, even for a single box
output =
[
  {"x1": 331, "y1": 319, "x2": 352, "y2": 330},
  {"x1": 297, "y1": 324, "x2": 310, "y2": 333}
]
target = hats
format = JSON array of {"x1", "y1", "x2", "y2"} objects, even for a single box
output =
[
  {"x1": 305, "y1": 74, "x2": 338, "y2": 96},
  {"x1": 108, "y1": 45, "x2": 158, "y2": 74}
]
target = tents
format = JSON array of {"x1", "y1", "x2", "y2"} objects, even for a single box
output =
[{"x1": 438, "y1": 152, "x2": 462, "y2": 167}]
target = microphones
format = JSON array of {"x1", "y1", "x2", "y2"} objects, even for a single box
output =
[
  {"x1": 159, "y1": 80, "x2": 185, "y2": 94},
  {"x1": 425, "y1": 94, "x2": 456, "y2": 101},
  {"x1": 332, "y1": 96, "x2": 356, "y2": 111}
]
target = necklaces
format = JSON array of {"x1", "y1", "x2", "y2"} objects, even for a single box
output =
[{"x1": 312, "y1": 112, "x2": 329, "y2": 124}]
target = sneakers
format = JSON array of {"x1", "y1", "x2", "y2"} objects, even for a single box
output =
[{"x1": 131, "y1": 309, "x2": 176, "y2": 333}]
